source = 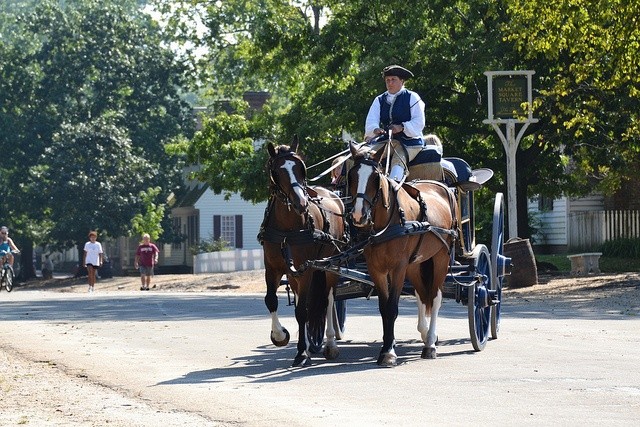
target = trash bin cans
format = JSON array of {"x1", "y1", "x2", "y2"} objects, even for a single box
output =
[{"x1": 503, "y1": 234, "x2": 541, "y2": 293}]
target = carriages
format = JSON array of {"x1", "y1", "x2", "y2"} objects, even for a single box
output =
[{"x1": 256, "y1": 126, "x2": 513, "y2": 368}]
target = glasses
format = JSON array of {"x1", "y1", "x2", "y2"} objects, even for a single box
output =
[{"x1": 0, "y1": 231, "x2": 8, "y2": 234}]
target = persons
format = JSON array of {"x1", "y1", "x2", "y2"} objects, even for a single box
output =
[
  {"x1": 82, "y1": 231, "x2": 104, "y2": 295},
  {"x1": 329, "y1": 156, "x2": 355, "y2": 185},
  {"x1": 362, "y1": 65, "x2": 427, "y2": 184},
  {"x1": 0, "y1": 225, "x2": 22, "y2": 287},
  {"x1": 134, "y1": 234, "x2": 161, "y2": 290}
]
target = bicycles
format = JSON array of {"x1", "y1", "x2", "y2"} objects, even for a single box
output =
[{"x1": 0, "y1": 249, "x2": 18, "y2": 292}]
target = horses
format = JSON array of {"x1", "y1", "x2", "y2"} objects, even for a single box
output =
[
  {"x1": 257, "y1": 132, "x2": 350, "y2": 369},
  {"x1": 338, "y1": 137, "x2": 477, "y2": 370}
]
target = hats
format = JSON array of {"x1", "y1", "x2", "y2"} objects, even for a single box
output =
[{"x1": 383, "y1": 64, "x2": 414, "y2": 80}]
